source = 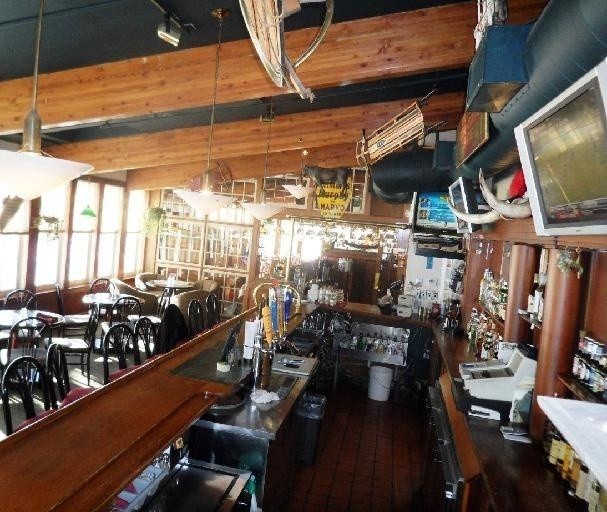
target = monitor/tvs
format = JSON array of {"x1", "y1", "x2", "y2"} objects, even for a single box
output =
[
  {"x1": 411, "y1": 190, "x2": 457, "y2": 238},
  {"x1": 448, "y1": 176, "x2": 482, "y2": 233},
  {"x1": 513, "y1": 58, "x2": 607, "y2": 237}
]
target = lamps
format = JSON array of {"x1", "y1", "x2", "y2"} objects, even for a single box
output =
[
  {"x1": 174, "y1": 1, "x2": 238, "y2": 219},
  {"x1": 79, "y1": 175, "x2": 98, "y2": 217},
  {"x1": 0, "y1": 0, "x2": 95, "y2": 201},
  {"x1": 282, "y1": 156, "x2": 314, "y2": 201},
  {"x1": 239, "y1": 99, "x2": 285, "y2": 223}
]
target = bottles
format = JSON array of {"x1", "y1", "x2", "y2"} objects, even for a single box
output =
[
  {"x1": 526, "y1": 271, "x2": 546, "y2": 324},
  {"x1": 544, "y1": 418, "x2": 607, "y2": 511},
  {"x1": 573, "y1": 337, "x2": 607, "y2": 396},
  {"x1": 466, "y1": 308, "x2": 501, "y2": 359},
  {"x1": 480, "y1": 267, "x2": 512, "y2": 320},
  {"x1": 442, "y1": 302, "x2": 462, "y2": 336}
]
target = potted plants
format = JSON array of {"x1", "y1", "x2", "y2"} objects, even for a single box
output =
[
  {"x1": 27, "y1": 212, "x2": 62, "y2": 240},
  {"x1": 140, "y1": 206, "x2": 170, "y2": 238}
]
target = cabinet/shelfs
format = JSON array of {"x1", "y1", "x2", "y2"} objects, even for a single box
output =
[
  {"x1": 260, "y1": 166, "x2": 373, "y2": 216},
  {"x1": 150, "y1": 175, "x2": 252, "y2": 314}
]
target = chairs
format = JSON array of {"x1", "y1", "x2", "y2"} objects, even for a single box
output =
[{"x1": 0, "y1": 266, "x2": 227, "y2": 438}]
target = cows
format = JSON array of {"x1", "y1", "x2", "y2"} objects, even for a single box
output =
[{"x1": 302, "y1": 165, "x2": 351, "y2": 196}]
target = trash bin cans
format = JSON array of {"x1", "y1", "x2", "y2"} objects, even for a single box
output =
[
  {"x1": 368, "y1": 362, "x2": 395, "y2": 402},
  {"x1": 290, "y1": 391, "x2": 328, "y2": 467}
]
